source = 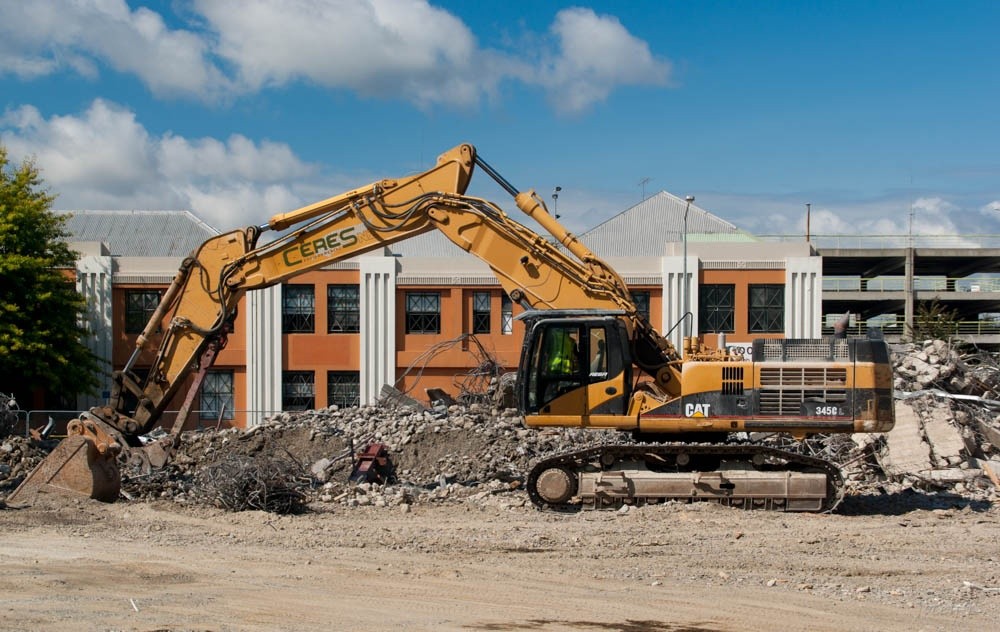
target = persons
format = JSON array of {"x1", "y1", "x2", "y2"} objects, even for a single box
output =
[{"x1": 541, "y1": 328, "x2": 578, "y2": 379}]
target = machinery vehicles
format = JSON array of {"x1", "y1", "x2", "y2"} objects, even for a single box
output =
[{"x1": 1, "y1": 143, "x2": 898, "y2": 515}]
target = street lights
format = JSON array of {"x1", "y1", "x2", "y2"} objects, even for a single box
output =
[
  {"x1": 551, "y1": 185, "x2": 563, "y2": 248},
  {"x1": 683, "y1": 194, "x2": 695, "y2": 343}
]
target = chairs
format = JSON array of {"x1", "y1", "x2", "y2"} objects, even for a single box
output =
[{"x1": 590, "y1": 339, "x2": 604, "y2": 372}]
